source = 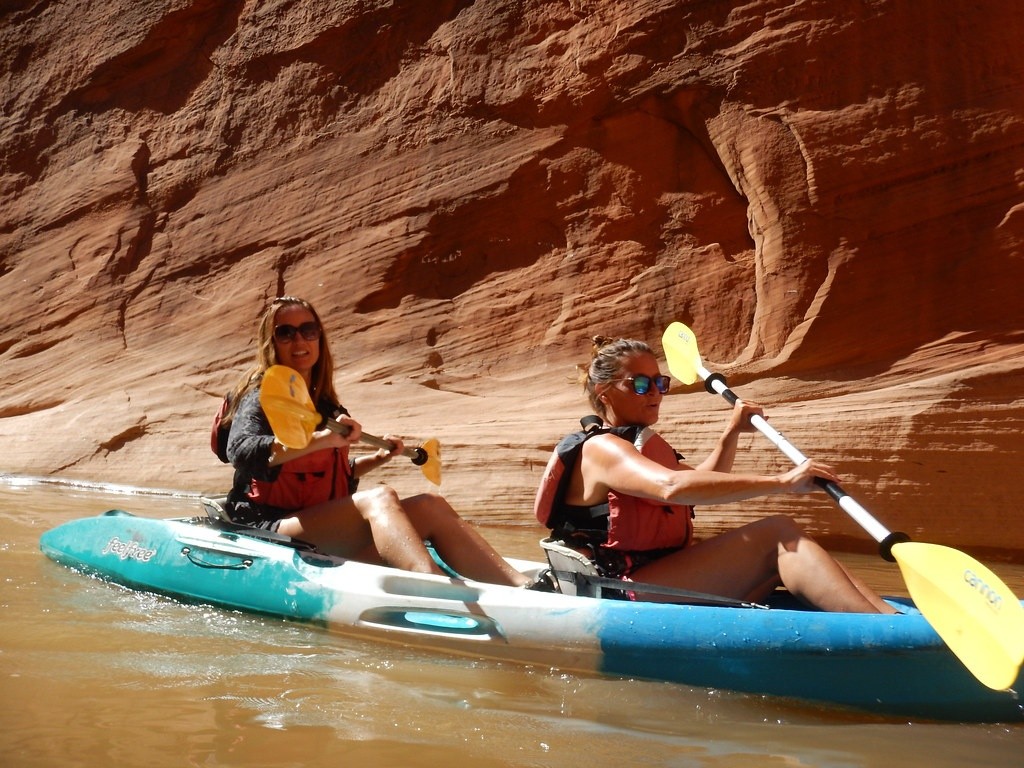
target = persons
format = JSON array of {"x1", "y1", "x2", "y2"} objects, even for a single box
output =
[
  {"x1": 535, "y1": 335, "x2": 906, "y2": 614},
  {"x1": 226, "y1": 296, "x2": 536, "y2": 588}
]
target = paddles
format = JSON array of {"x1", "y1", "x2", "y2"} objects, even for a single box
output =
[
  {"x1": 257, "y1": 363, "x2": 444, "y2": 487},
  {"x1": 659, "y1": 321, "x2": 1024, "y2": 693}
]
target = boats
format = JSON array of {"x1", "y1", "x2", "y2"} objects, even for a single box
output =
[{"x1": 39, "y1": 504, "x2": 1024, "y2": 724}]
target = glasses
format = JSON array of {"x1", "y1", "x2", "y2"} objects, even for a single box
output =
[
  {"x1": 273, "y1": 321, "x2": 321, "y2": 344},
  {"x1": 606, "y1": 373, "x2": 671, "y2": 397}
]
